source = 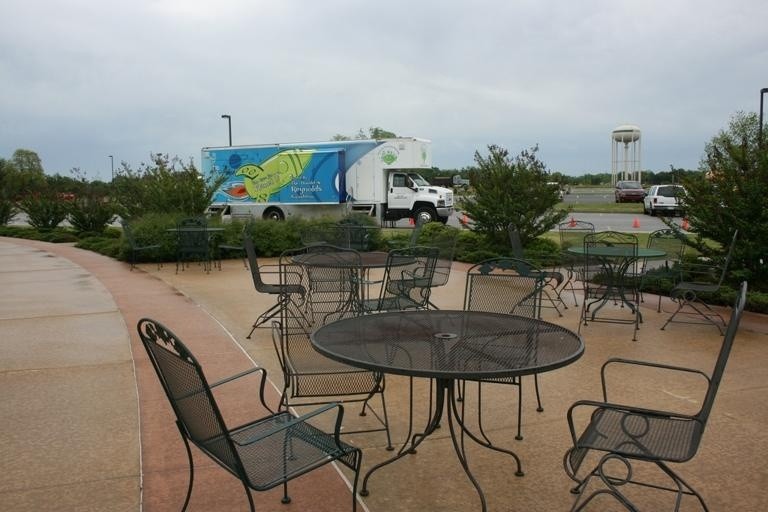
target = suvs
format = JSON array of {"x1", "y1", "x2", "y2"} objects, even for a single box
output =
[{"x1": 641, "y1": 185, "x2": 689, "y2": 218}]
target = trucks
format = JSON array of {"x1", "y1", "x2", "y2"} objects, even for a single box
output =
[
  {"x1": 199, "y1": 136, "x2": 456, "y2": 228},
  {"x1": 430, "y1": 170, "x2": 471, "y2": 188}
]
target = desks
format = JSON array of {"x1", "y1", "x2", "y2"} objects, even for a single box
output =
[{"x1": 309, "y1": 310, "x2": 586, "y2": 507}]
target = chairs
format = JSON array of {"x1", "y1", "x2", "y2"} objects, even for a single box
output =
[
  {"x1": 230, "y1": 219, "x2": 464, "y2": 340},
  {"x1": 489, "y1": 212, "x2": 744, "y2": 340},
  {"x1": 430, "y1": 256, "x2": 546, "y2": 440},
  {"x1": 268, "y1": 243, "x2": 393, "y2": 456},
  {"x1": 133, "y1": 314, "x2": 363, "y2": 510},
  {"x1": 561, "y1": 279, "x2": 751, "y2": 509}
]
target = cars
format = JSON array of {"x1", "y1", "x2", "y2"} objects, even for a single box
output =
[
  {"x1": 544, "y1": 181, "x2": 564, "y2": 202},
  {"x1": 613, "y1": 180, "x2": 647, "y2": 204}
]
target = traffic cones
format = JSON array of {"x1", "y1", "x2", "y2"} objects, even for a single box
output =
[
  {"x1": 462, "y1": 216, "x2": 468, "y2": 226},
  {"x1": 631, "y1": 217, "x2": 641, "y2": 228},
  {"x1": 680, "y1": 220, "x2": 689, "y2": 231},
  {"x1": 567, "y1": 217, "x2": 577, "y2": 228},
  {"x1": 409, "y1": 218, "x2": 414, "y2": 226}
]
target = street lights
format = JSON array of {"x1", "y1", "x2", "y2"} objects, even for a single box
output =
[
  {"x1": 107, "y1": 154, "x2": 115, "y2": 182},
  {"x1": 669, "y1": 163, "x2": 674, "y2": 184},
  {"x1": 758, "y1": 86, "x2": 767, "y2": 140},
  {"x1": 220, "y1": 115, "x2": 233, "y2": 146}
]
